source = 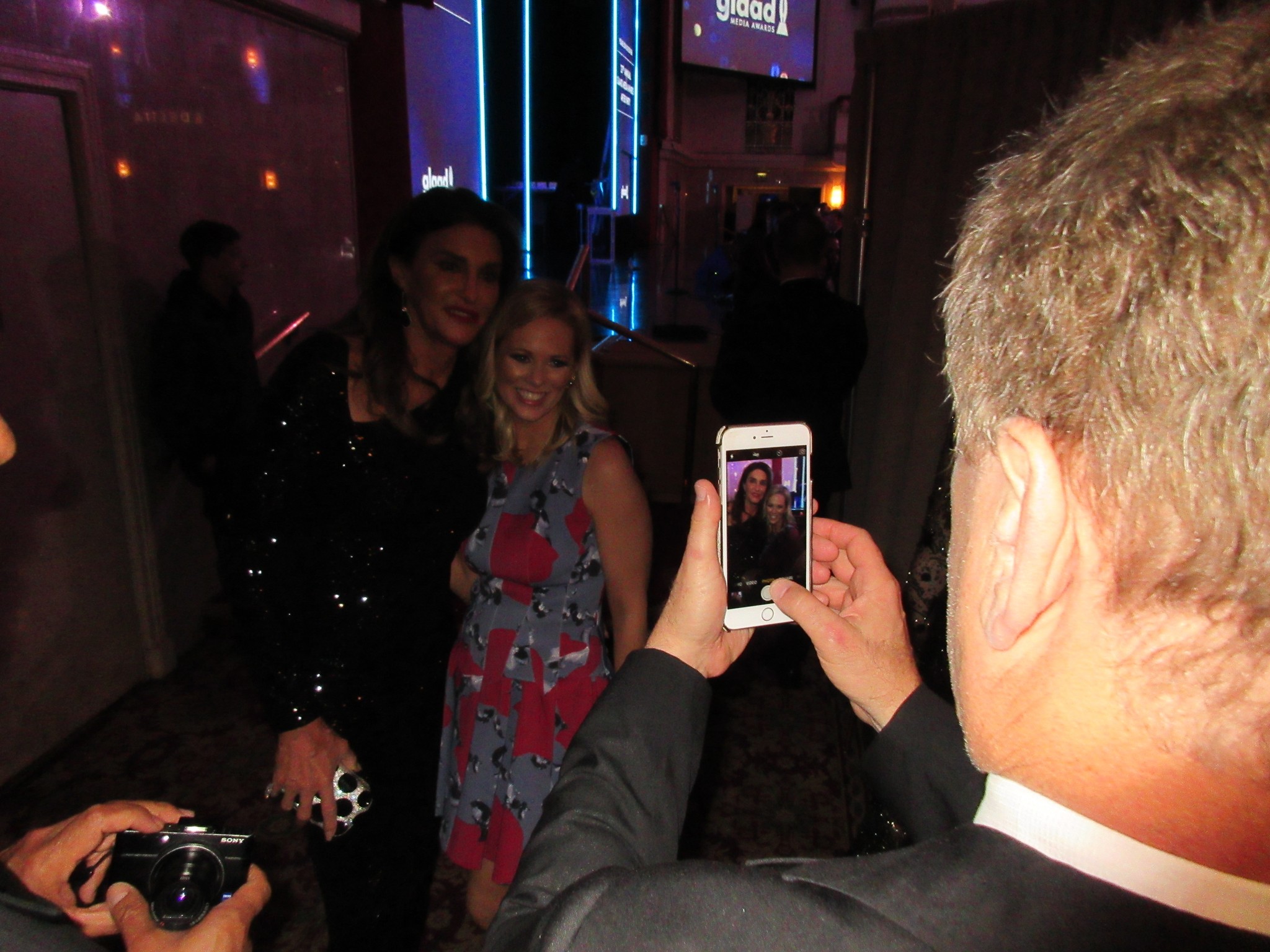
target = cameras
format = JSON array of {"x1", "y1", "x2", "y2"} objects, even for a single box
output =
[{"x1": 114, "y1": 817, "x2": 258, "y2": 935}]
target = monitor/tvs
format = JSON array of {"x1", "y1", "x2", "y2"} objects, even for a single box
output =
[{"x1": 675, "y1": 0, "x2": 819, "y2": 93}]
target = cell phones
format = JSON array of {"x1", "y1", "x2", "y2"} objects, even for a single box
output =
[{"x1": 715, "y1": 419, "x2": 813, "y2": 634}]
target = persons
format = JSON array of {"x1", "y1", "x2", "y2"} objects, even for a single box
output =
[
  {"x1": 273, "y1": 180, "x2": 512, "y2": 951},
  {"x1": 144, "y1": 215, "x2": 277, "y2": 635},
  {"x1": 1, "y1": 412, "x2": 275, "y2": 952},
  {"x1": 484, "y1": 6, "x2": 1270, "y2": 952},
  {"x1": 436, "y1": 279, "x2": 653, "y2": 952},
  {"x1": 709, "y1": 203, "x2": 870, "y2": 513},
  {"x1": 725, "y1": 462, "x2": 772, "y2": 591},
  {"x1": 733, "y1": 482, "x2": 802, "y2": 604}
]
